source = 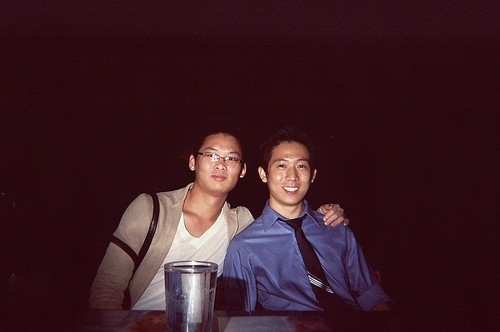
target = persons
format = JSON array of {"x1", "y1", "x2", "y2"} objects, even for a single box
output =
[
  {"x1": 88, "y1": 120, "x2": 350, "y2": 312},
  {"x1": 222, "y1": 125, "x2": 394, "y2": 312}
]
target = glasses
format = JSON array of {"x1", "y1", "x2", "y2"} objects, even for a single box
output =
[{"x1": 197, "y1": 152, "x2": 244, "y2": 166}]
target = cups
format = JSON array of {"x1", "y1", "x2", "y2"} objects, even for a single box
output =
[{"x1": 163, "y1": 260, "x2": 219, "y2": 332}]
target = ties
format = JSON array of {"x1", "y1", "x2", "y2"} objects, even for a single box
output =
[{"x1": 277, "y1": 214, "x2": 345, "y2": 313}]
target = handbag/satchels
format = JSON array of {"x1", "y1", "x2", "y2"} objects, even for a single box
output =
[{"x1": 121, "y1": 288, "x2": 132, "y2": 310}]
